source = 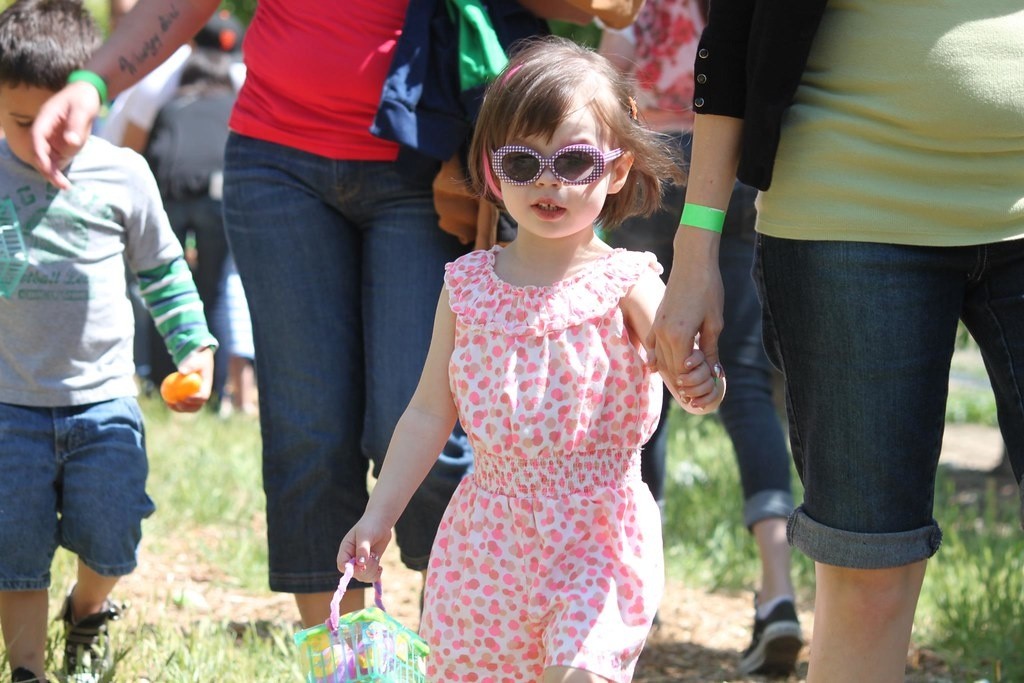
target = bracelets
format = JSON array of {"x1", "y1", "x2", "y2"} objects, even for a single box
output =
[
  {"x1": 679, "y1": 202, "x2": 727, "y2": 235},
  {"x1": 69, "y1": 71, "x2": 112, "y2": 115}
]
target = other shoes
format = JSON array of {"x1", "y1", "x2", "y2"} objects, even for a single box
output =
[
  {"x1": 61, "y1": 579, "x2": 112, "y2": 683},
  {"x1": 740, "y1": 592, "x2": 803, "y2": 678}
]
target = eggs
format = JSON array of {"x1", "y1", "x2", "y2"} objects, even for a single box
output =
[{"x1": 160, "y1": 371, "x2": 201, "y2": 403}]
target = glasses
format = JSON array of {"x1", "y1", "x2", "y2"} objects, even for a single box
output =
[{"x1": 488, "y1": 144, "x2": 625, "y2": 185}]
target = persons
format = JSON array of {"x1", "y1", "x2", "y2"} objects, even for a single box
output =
[
  {"x1": 335, "y1": 34, "x2": 728, "y2": 682},
  {"x1": 0, "y1": 0, "x2": 219, "y2": 682},
  {"x1": 492, "y1": 0, "x2": 1024, "y2": 682},
  {"x1": 91, "y1": 10, "x2": 264, "y2": 413},
  {"x1": 29, "y1": 0, "x2": 645, "y2": 629}
]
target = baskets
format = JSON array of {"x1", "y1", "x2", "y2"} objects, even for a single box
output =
[{"x1": 295, "y1": 556, "x2": 430, "y2": 683}]
